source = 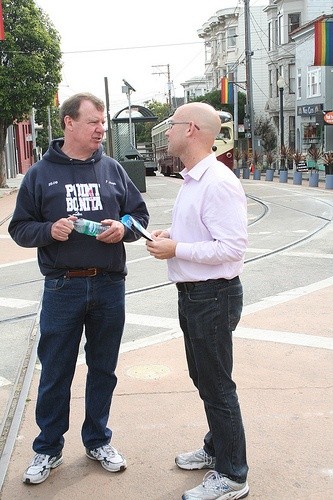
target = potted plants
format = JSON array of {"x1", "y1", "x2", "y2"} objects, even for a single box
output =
[{"x1": 233, "y1": 145, "x2": 333, "y2": 189}]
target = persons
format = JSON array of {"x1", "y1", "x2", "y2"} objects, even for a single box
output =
[
  {"x1": 8, "y1": 92, "x2": 149, "y2": 484},
  {"x1": 147, "y1": 102, "x2": 250, "y2": 500}
]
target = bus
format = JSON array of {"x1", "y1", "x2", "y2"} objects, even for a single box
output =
[{"x1": 150, "y1": 109, "x2": 234, "y2": 179}]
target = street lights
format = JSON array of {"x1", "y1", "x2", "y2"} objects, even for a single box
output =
[
  {"x1": 276, "y1": 75, "x2": 289, "y2": 175},
  {"x1": 121, "y1": 79, "x2": 137, "y2": 149}
]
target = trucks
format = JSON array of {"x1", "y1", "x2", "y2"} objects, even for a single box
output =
[{"x1": 134, "y1": 142, "x2": 158, "y2": 175}]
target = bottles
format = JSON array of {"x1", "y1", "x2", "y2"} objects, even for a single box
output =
[{"x1": 67, "y1": 218, "x2": 112, "y2": 237}]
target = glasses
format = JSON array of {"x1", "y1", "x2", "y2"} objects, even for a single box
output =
[{"x1": 167, "y1": 120, "x2": 200, "y2": 131}]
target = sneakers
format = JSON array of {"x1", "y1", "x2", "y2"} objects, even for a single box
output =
[
  {"x1": 22, "y1": 451, "x2": 63, "y2": 484},
  {"x1": 175, "y1": 447, "x2": 217, "y2": 471},
  {"x1": 181, "y1": 470, "x2": 249, "y2": 500},
  {"x1": 85, "y1": 443, "x2": 127, "y2": 472}
]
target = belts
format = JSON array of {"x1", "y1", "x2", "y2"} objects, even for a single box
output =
[
  {"x1": 176, "y1": 279, "x2": 225, "y2": 291},
  {"x1": 68, "y1": 268, "x2": 101, "y2": 276}
]
